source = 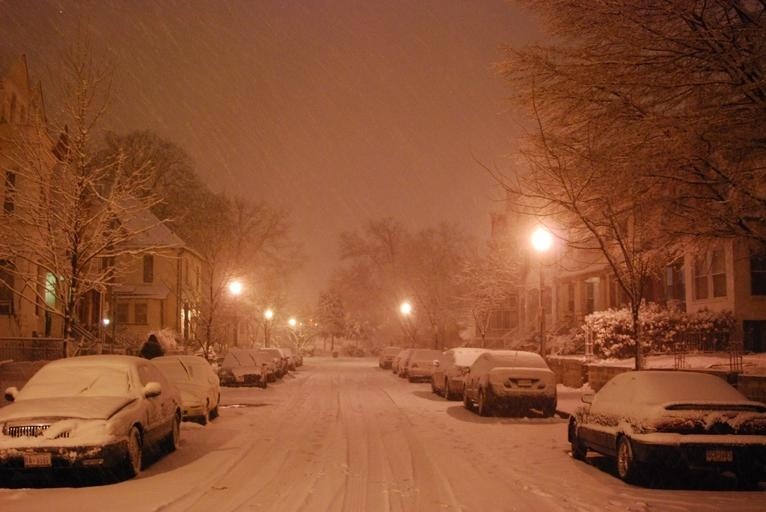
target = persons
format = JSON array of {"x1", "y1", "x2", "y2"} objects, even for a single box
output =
[{"x1": 139, "y1": 334, "x2": 162, "y2": 360}]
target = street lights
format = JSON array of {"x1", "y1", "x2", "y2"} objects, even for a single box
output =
[
  {"x1": 400, "y1": 301, "x2": 411, "y2": 342},
  {"x1": 263, "y1": 307, "x2": 273, "y2": 348},
  {"x1": 229, "y1": 280, "x2": 240, "y2": 347},
  {"x1": 531, "y1": 224, "x2": 553, "y2": 351},
  {"x1": 287, "y1": 318, "x2": 296, "y2": 337},
  {"x1": 102, "y1": 318, "x2": 110, "y2": 343}
]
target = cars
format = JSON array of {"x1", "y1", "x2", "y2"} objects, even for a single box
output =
[
  {"x1": 146, "y1": 353, "x2": 223, "y2": 426},
  {"x1": 376, "y1": 345, "x2": 443, "y2": 381},
  {"x1": 0, "y1": 352, "x2": 186, "y2": 484},
  {"x1": 426, "y1": 346, "x2": 491, "y2": 398},
  {"x1": 458, "y1": 349, "x2": 560, "y2": 419},
  {"x1": 566, "y1": 368, "x2": 766, "y2": 484},
  {"x1": 217, "y1": 345, "x2": 303, "y2": 389}
]
